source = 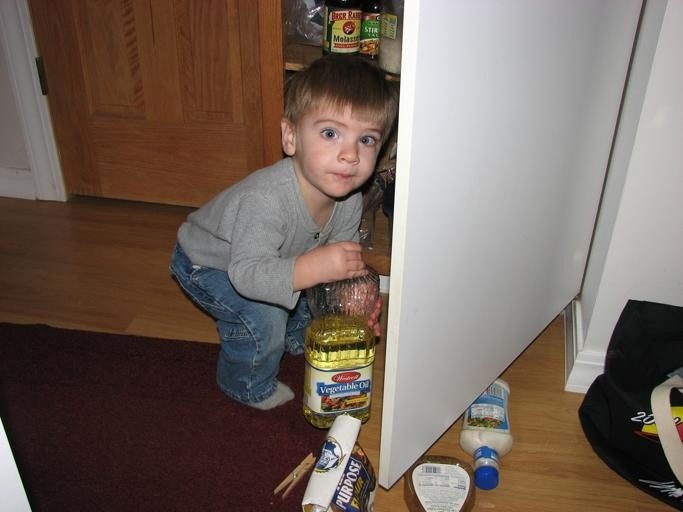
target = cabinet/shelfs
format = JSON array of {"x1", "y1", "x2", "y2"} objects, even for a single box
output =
[{"x1": 257, "y1": 0, "x2": 404, "y2": 275}]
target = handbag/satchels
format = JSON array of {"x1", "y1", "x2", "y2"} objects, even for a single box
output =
[{"x1": 578, "y1": 298, "x2": 683, "y2": 511}]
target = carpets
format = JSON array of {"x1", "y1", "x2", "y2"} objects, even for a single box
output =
[{"x1": 0, "y1": 321, "x2": 329, "y2": 511}]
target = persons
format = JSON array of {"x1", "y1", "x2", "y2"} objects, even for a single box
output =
[{"x1": 168, "y1": 51, "x2": 400, "y2": 410}]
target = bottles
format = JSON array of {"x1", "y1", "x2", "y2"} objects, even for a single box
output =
[
  {"x1": 323, "y1": 0, "x2": 383, "y2": 64},
  {"x1": 456, "y1": 378, "x2": 513, "y2": 497}
]
